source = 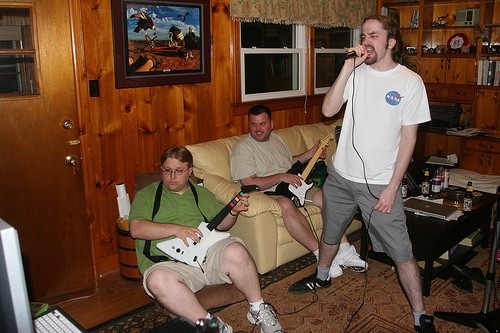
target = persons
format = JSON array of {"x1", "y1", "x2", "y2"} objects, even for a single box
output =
[
  {"x1": 129, "y1": 144, "x2": 285, "y2": 333},
  {"x1": 184, "y1": 26, "x2": 195, "y2": 58},
  {"x1": 288, "y1": 14, "x2": 436, "y2": 333},
  {"x1": 230, "y1": 105, "x2": 368, "y2": 278}
]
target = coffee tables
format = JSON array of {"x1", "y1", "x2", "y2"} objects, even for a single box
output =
[{"x1": 353, "y1": 185, "x2": 497, "y2": 299}]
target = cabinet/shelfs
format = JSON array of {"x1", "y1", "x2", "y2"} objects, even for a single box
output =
[{"x1": 376, "y1": 0, "x2": 500, "y2": 174}]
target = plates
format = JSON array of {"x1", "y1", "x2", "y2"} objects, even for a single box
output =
[{"x1": 444, "y1": 191, "x2": 465, "y2": 204}]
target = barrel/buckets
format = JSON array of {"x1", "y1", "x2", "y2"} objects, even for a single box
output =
[{"x1": 117, "y1": 228, "x2": 143, "y2": 280}]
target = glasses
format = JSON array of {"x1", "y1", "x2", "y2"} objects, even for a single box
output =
[{"x1": 160, "y1": 167, "x2": 190, "y2": 175}]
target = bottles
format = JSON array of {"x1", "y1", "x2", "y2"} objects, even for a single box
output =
[
  {"x1": 402, "y1": 174, "x2": 408, "y2": 199},
  {"x1": 463, "y1": 181, "x2": 473, "y2": 214},
  {"x1": 431, "y1": 165, "x2": 450, "y2": 195},
  {"x1": 421, "y1": 168, "x2": 430, "y2": 196}
]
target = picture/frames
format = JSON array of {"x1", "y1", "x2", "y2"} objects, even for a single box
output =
[{"x1": 110, "y1": 0, "x2": 212, "y2": 89}]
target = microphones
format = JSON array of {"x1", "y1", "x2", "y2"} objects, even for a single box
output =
[{"x1": 339, "y1": 51, "x2": 356, "y2": 62}]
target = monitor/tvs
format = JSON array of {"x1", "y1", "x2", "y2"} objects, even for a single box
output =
[{"x1": 0, "y1": 218, "x2": 35, "y2": 333}]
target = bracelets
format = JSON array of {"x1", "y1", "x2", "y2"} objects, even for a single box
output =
[{"x1": 230, "y1": 212, "x2": 239, "y2": 216}]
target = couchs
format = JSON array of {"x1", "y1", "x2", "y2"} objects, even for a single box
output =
[{"x1": 186, "y1": 118, "x2": 362, "y2": 273}]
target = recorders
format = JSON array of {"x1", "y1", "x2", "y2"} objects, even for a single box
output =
[{"x1": 455, "y1": 8, "x2": 480, "y2": 26}]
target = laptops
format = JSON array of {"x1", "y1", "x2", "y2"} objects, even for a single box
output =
[{"x1": 403, "y1": 198, "x2": 458, "y2": 219}]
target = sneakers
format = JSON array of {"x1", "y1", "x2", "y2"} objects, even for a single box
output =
[
  {"x1": 215, "y1": 315, "x2": 234, "y2": 333},
  {"x1": 313, "y1": 243, "x2": 369, "y2": 279},
  {"x1": 413, "y1": 313, "x2": 436, "y2": 333},
  {"x1": 288, "y1": 273, "x2": 332, "y2": 294},
  {"x1": 246, "y1": 303, "x2": 283, "y2": 333}
]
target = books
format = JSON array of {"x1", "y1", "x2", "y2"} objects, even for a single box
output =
[{"x1": 477, "y1": 60, "x2": 500, "y2": 86}]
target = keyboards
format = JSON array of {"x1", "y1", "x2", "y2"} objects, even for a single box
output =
[{"x1": 33, "y1": 309, "x2": 82, "y2": 333}]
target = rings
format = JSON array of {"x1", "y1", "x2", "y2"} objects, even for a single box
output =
[{"x1": 183, "y1": 238, "x2": 185, "y2": 240}]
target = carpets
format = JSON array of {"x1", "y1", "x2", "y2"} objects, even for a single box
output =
[{"x1": 87, "y1": 226, "x2": 500, "y2": 333}]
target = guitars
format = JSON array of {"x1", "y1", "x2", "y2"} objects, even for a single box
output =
[
  {"x1": 156, "y1": 184, "x2": 261, "y2": 269},
  {"x1": 275, "y1": 132, "x2": 335, "y2": 207}
]
